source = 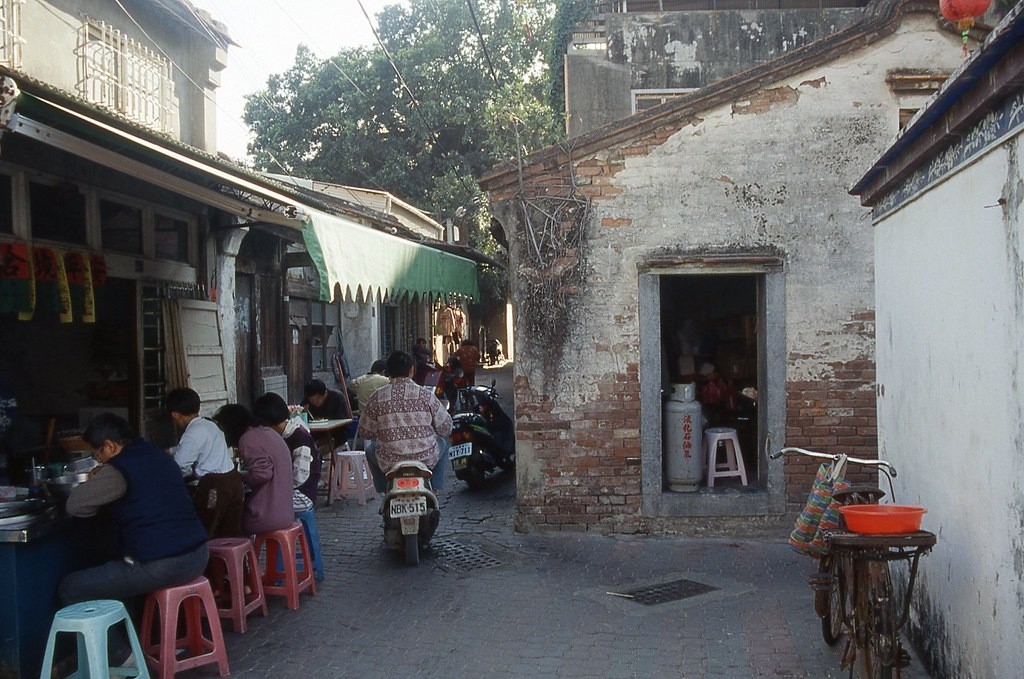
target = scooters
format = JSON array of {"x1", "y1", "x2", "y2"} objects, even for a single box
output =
[
  {"x1": 448, "y1": 367, "x2": 515, "y2": 489},
  {"x1": 378, "y1": 398, "x2": 450, "y2": 566}
]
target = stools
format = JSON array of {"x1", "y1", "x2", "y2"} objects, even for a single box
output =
[
  {"x1": 702, "y1": 427, "x2": 748, "y2": 487},
  {"x1": 249, "y1": 522, "x2": 316, "y2": 610},
  {"x1": 40, "y1": 599, "x2": 152, "y2": 679},
  {"x1": 328, "y1": 441, "x2": 351, "y2": 489},
  {"x1": 199, "y1": 537, "x2": 268, "y2": 633},
  {"x1": 329, "y1": 451, "x2": 376, "y2": 505},
  {"x1": 137, "y1": 576, "x2": 230, "y2": 679},
  {"x1": 275, "y1": 506, "x2": 325, "y2": 584}
]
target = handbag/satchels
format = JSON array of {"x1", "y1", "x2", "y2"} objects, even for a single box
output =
[{"x1": 786, "y1": 454, "x2": 852, "y2": 559}]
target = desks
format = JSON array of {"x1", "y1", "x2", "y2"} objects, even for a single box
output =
[{"x1": 307, "y1": 418, "x2": 352, "y2": 483}]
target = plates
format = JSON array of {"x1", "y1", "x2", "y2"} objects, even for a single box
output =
[{"x1": 309, "y1": 420, "x2": 329, "y2": 423}]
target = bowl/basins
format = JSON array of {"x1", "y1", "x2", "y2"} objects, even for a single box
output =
[
  {"x1": 838, "y1": 506, "x2": 927, "y2": 534},
  {"x1": 47, "y1": 472, "x2": 96, "y2": 504}
]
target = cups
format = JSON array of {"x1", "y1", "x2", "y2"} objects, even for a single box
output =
[{"x1": 28, "y1": 467, "x2": 47, "y2": 498}]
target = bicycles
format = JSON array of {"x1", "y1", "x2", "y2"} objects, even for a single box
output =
[{"x1": 771, "y1": 447, "x2": 938, "y2": 679}]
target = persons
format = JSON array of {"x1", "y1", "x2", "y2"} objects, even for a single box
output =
[
  {"x1": 211, "y1": 404, "x2": 294, "y2": 532},
  {"x1": 411, "y1": 338, "x2": 480, "y2": 386},
  {"x1": 59, "y1": 413, "x2": 209, "y2": 679},
  {"x1": 164, "y1": 387, "x2": 251, "y2": 597},
  {"x1": 301, "y1": 378, "x2": 351, "y2": 456},
  {"x1": 358, "y1": 351, "x2": 452, "y2": 494},
  {"x1": 253, "y1": 392, "x2": 320, "y2": 511},
  {"x1": 0, "y1": 373, "x2": 17, "y2": 488},
  {"x1": 351, "y1": 360, "x2": 390, "y2": 421}
]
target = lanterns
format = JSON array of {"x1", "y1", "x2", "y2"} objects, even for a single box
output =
[{"x1": 939, "y1": 0, "x2": 991, "y2": 56}]
